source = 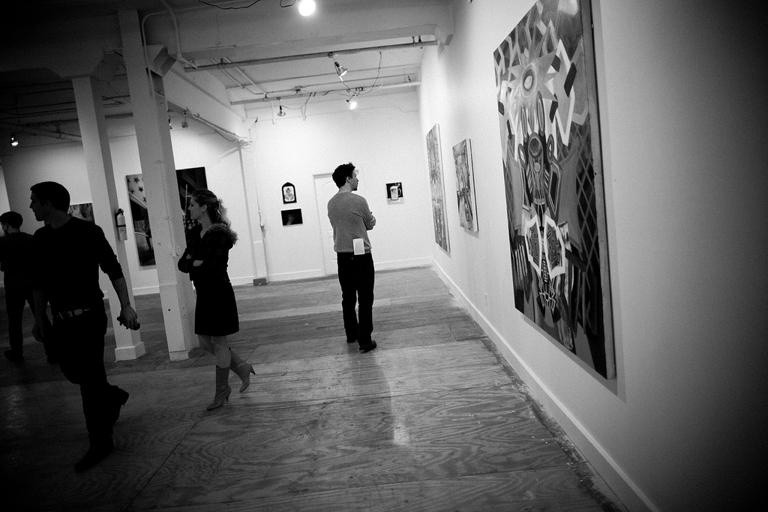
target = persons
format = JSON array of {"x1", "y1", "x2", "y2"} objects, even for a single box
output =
[
  {"x1": 0, "y1": 211, "x2": 35, "y2": 365},
  {"x1": 177, "y1": 189, "x2": 257, "y2": 411},
  {"x1": 327, "y1": 161, "x2": 377, "y2": 353},
  {"x1": 29, "y1": 181, "x2": 140, "y2": 473}
]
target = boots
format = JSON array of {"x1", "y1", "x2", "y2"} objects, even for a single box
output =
[
  {"x1": 206, "y1": 365, "x2": 231, "y2": 410},
  {"x1": 228, "y1": 348, "x2": 256, "y2": 392}
]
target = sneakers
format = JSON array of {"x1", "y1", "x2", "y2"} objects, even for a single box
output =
[
  {"x1": 76, "y1": 442, "x2": 112, "y2": 474},
  {"x1": 108, "y1": 387, "x2": 129, "y2": 426},
  {"x1": 359, "y1": 341, "x2": 376, "y2": 350},
  {"x1": 344, "y1": 334, "x2": 358, "y2": 343}
]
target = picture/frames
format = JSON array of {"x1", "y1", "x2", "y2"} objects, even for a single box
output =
[{"x1": 282, "y1": 182, "x2": 296, "y2": 203}]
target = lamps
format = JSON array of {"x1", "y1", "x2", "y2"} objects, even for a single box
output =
[
  {"x1": 334, "y1": 61, "x2": 348, "y2": 77},
  {"x1": 277, "y1": 106, "x2": 287, "y2": 118}
]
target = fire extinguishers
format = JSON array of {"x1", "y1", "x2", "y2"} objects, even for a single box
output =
[{"x1": 115, "y1": 208, "x2": 128, "y2": 241}]
